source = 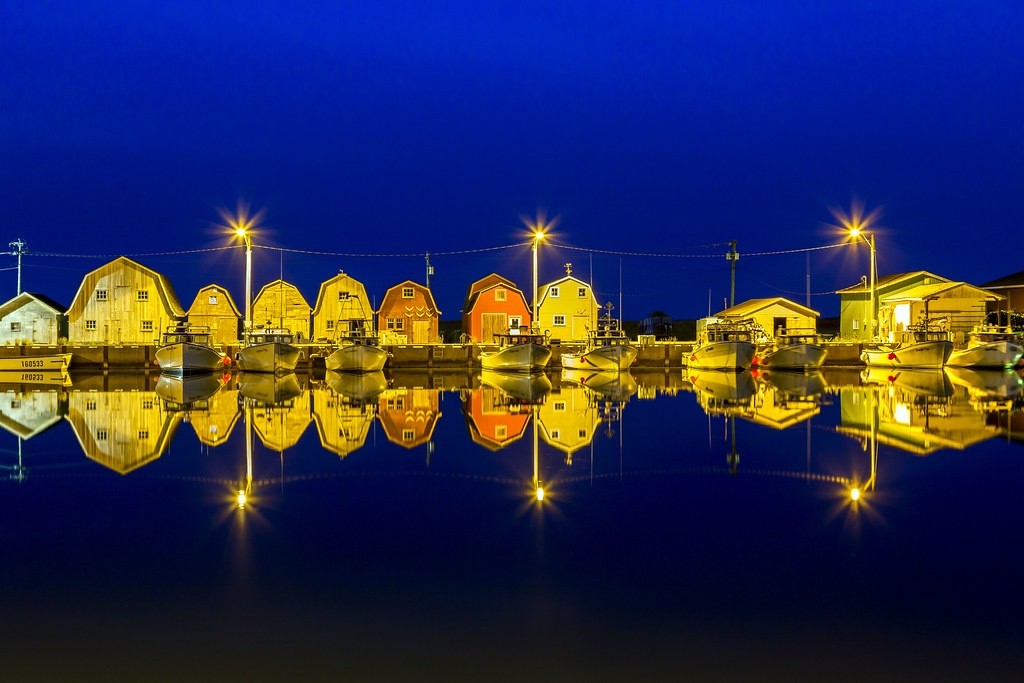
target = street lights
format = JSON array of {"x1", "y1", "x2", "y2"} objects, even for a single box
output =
[
  {"x1": 851, "y1": 228, "x2": 876, "y2": 340},
  {"x1": 532, "y1": 233, "x2": 544, "y2": 333},
  {"x1": 235, "y1": 228, "x2": 252, "y2": 343}
]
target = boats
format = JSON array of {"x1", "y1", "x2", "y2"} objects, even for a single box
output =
[
  {"x1": 682, "y1": 365, "x2": 757, "y2": 446},
  {"x1": 561, "y1": 369, "x2": 637, "y2": 446},
  {"x1": 310, "y1": 291, "x2": 394, "y2": 372},
  {"x1": 325, "y1": 368, "x2": 389, "y2": 446},
  {"x1": 860, "y1": 294, "x2": 957, "y2": 369},
  {"x1": 0, "y1": 352, "x2": 75, "y2": 371},
  {"x1": 562, "y1": 300, "x2": 637, "y2": 372},
  {"x1": 0, "y1": 369, "x2": 304, "y2": 428},
  {"x1": 759, "y1": 321, "x2": 826, "y2": 367},
  {"x1": 682, "y1": 315, "x2": 757, "y2": 371},
  {"x1": 753, "y1": 367, "x2": 829, "y2": 407},
  {"x1": 862, "y1": 367, "x2": 1024, "y2": 429},
  {"x1": 155, "y1": 325, "x2": 225, "y2": 371},
  {"x1": 946, "y1": 304, "x2": 1024, "y2": 367},
  {"x1": 480, "y1": 368, "x2": 552, "y2": 410},
  {"x1": 235, "y1": 328, "x2": 303, "y2": 372},
  {"x1": 478, "y1": 326, "x2": 553, "y2": 370}
]
